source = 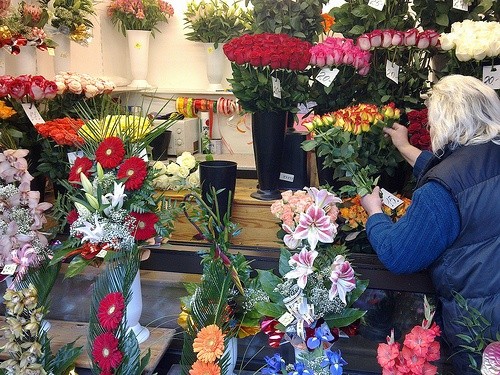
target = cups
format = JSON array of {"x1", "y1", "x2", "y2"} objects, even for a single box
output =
[
  {"x1": 199, "y1": 160, "x2": 237, "y2": 240},
  {"x1": 112, "y1": 98, "x2": 121, "y2": 104}
]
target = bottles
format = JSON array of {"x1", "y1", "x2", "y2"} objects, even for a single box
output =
[
  {"x1": 122, "y1": 107, "x2": 131, "y2": 114},
  {"x1": 128, "y1": 106, "x2": 141, "y2": 115}
]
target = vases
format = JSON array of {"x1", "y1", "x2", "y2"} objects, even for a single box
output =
[
  {"x1": 250, "y1": 106, "x2": 287, "y2": 200},
  {"x1": 105, "y1": 262, "x2": 150, "y2": 345},
  {"x1": 188, "y1": 160, "x2": 239, "y2": 243},
  {"x1": 125, "y1": 29, "x2": 152, "y2": 87},
  {"x1": 313, "y1": 109, "x2": 350, "y2": 192},
  {"x1": 4, "y1": 259, "x2": 52, "y2": 334},
  {"x1": 52, "y1": 35, "x2": 73, "y2": 77},
  {"x1": 221, "y1": 324, "x2": 238, "y2": 375},
  {"x1": 147, "y1": 130, "x2": 172, "y2": 163},
  {"x1": 203, "y1": 42, "x2": 230, "y2": 91},
  {"x1": 4, "y1": 46, "x2": 41, "y2": 80},
  {"x1": 52, "y1": 177, "x2": 68, "y2": 200},
  {"x1": 31, "y1": 175, "x2": 47, "y2": 204}
]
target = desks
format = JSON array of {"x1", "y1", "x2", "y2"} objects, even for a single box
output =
[{"x1": 153, "y1": 178, "x2": 286, "y2": 248}]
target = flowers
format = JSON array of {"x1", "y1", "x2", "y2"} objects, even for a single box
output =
[{"x1": 0, "y1": 0, "x2": 500, "y2": 375}]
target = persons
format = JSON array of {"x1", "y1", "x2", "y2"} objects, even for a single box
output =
[{"x1": 360, "y1": 74, "x2": 500, "y2": 375}]
